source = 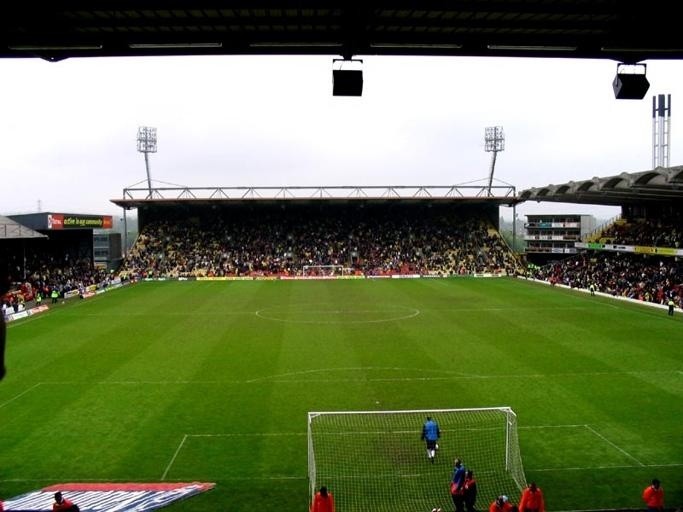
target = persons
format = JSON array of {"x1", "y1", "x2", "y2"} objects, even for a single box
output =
[
  {"x1": 314, "y1": 485, "x2": 335, "y2": 512},
  {"x1": 529, "y1": 201, "x2": 683, "y2": 316},
  {"x1": 420, "y1": 417, "x2": 441, "y2": 464},
  {"x1": 643, "y1": 479, "x2": 666, "y2": 512},
  {"x1": 518, "y1": 482, "x2": 547, "y2": 512},
  {"x1": 489, "y1": 494, "x2": 512, "y2": 512},
  {"x1": 111, "y1": 201, "x2": 529, "y2": 280},
  {"x1": 452, "y1": 455, "x2": 465, "y2": 512},
  {"x1": 1, "y1": 247, "x2": 111, "y2": 315},
  {"x1": 52, "y1": 493, "x2": 72, "y2": 511},
  {"x1": 461, "y1": 470, "x2": 477, "y2": 512}
]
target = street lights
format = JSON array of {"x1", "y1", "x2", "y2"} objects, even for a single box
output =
[
  {"x1": 484, "y1": 125, "x2": 505, "y2": 195},
  {"x1": 138, "y1": 126, "x2": 158, "y2": 199}
]
